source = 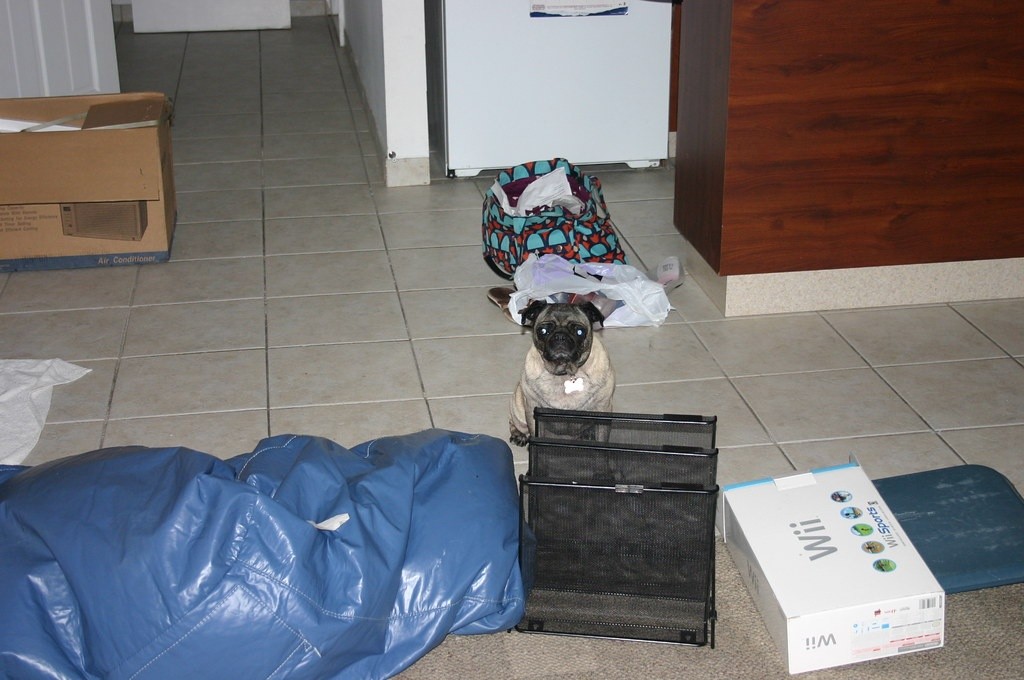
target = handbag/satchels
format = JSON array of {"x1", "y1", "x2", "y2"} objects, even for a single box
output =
[{"x1": 482, "y1": 158, "x2": 631, "y2": 282}]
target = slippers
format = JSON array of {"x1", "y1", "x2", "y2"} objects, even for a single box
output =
[{"x1": 487, "y1": 287, "x2": 534, "y2": 324}]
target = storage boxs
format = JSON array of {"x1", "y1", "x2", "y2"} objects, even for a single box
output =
[
  {"x1": 713, "y1": 449, "x2": 945, "y2": 676},
  {"x1": 0, "y1": 92, "x2": 178, "y2": 274}
]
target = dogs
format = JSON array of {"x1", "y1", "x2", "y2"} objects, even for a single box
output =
[{"x1": 509, "y1": 300, "x2": 617, "y2": 480}]
